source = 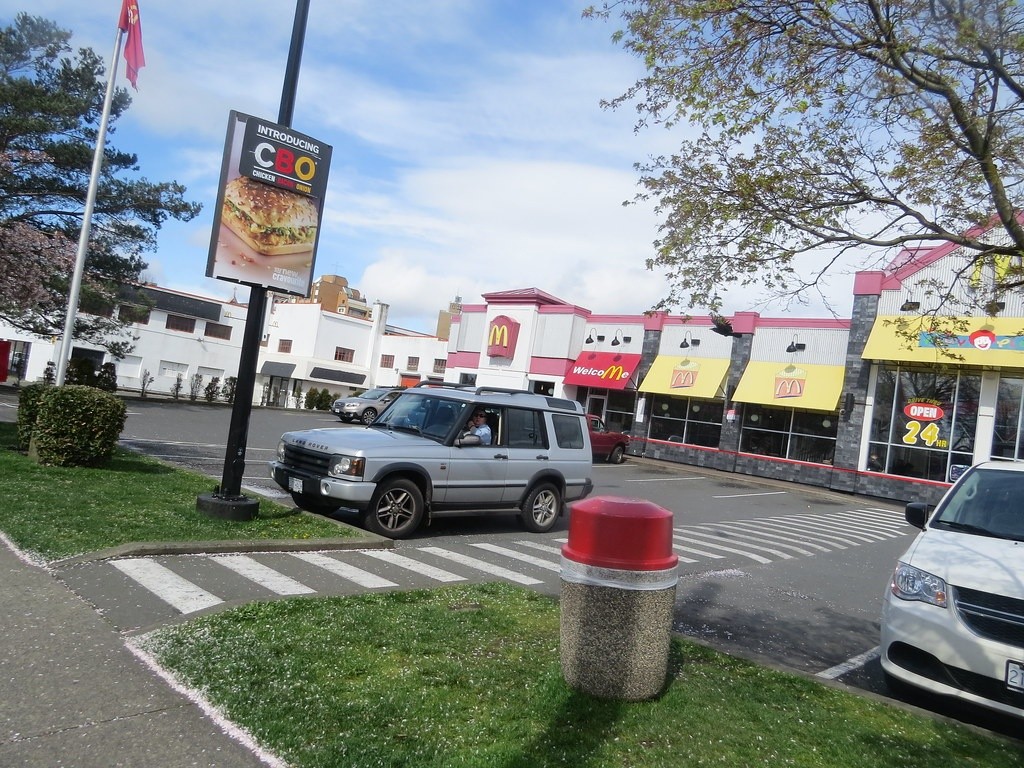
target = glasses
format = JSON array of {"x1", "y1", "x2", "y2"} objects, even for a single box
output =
[{"x1": 473, "y1": 414, "x2": 486, "y2": 417}]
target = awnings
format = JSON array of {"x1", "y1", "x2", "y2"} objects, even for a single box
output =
[
  {"x1": 638, "y1": 355, "x2": 731, "y2": 399},
  {"x1": 562, "y1": 351, "x2": 641, "y2": 390},
  {"x1": 861, "y1": 314, "x2": 1024, "y2": 367},
  {"x1": 731, "y1": 361, "x2": 845, "y2": 411}
]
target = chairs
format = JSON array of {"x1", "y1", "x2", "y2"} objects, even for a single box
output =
[
  {"x1": 426, "y1": 407, "x2": 453, "y2": 435},
  {"x1": 487, "y1": 411, "x2": 498, "y2": 445}
]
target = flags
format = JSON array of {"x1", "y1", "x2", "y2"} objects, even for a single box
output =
[{"x1": 119, "y1": 0, "x2": 146, "y2": 93}]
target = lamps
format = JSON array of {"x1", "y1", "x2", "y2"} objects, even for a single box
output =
[
  {"x1": 692, "y1": 405, "x2": 700, "y2": 412},
  {"x1": 751, "y1": 412, "x2": 758, "y2": 421},
  {"x1": 585, "y1": 327, "x2": 605, "y2": 343},
  {"x1": 822, "y1": 417, "x2": 831, "y2": 428},
  {"x1": 611, "y1": 329, "x2": 631, "y2": 346},
  {"x1": 662, "y1": 403, "x2": 669, "y2": 410},
  {"x1": 991, "y1": 285, "x2": 1006, "y2": 310},
  {"x1": 899, "y1": 290, "x2": 920, "y2": 311},
  {"x1": 679, "y1": 330, "x2": 700, "y2": 348},
  {"x1": 840, "y1": 391, "x2": 855, "y2": 422},
  {"x1": 547, "y1": 383, "x2": 554, "y2": 395},
  {"x1": 787, "y1": 333, "x2": 805, "y2": 352}
]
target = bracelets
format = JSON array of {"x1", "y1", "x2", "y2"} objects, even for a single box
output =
[{"x1": 469, "y1": 425, "x2": 474, "y2": 429}]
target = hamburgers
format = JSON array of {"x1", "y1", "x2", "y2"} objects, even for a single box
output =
[{"x1": 220, "y1": 175, "x2": 319, "y2": 256}]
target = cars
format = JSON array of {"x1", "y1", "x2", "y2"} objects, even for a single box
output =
[
  {"x1": 880, "y1": 460, "x2": 1024, "y2": 721},
  {"x1": 582, "y1": 412, "x2": 630, "y2": 464}
]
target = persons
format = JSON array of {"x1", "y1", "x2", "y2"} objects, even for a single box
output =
[
  {"x1": 463, "y1": 409, "x2": 491, "y2": 446},
  {"x1": 867, "y1": 453, "x2": 883, "y2": 472}
]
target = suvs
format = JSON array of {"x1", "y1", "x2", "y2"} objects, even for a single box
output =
[
  {"x1": 332, "y1": 385, "x2": 408, "y2": 425},
  {"x1": 269, "y1": 380, "x2": 595, "y2": 538}
]
